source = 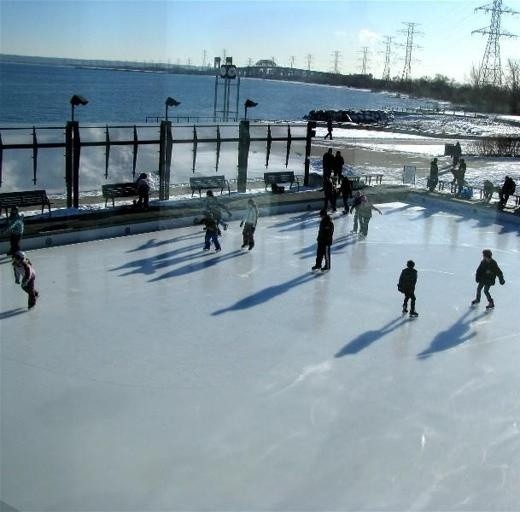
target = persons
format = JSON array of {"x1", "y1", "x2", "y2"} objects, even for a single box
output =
[
  {"x1": 199, "y1": 190, "x2": 260, "y2": 252},
  {"x1": 322, "y1": 146, "x2": 383, "y2": 237},
  {"x1": 324, "y1": 120, "x2": 334, "y2": 140},
  {"x1": 311, "y1": 208, "x2": 334, "y2": 271},
  {"x1": 397, "y1": 260, "x2": 419, "y2": 318},
  {"x1": 2, "y1": 206, "x2": 24, "y2": 256},
  {"x1": 427, "y1": 141, "x2": 516, "y2": 209},
  {"x1": 137, "y1": 173, "x2": 148, "y2": 204},
  {"x1": 472, "y1": 249, "x2": 505, "y2": 308},
  {"x1": 12, "y1": 251, "x2": 39, "y2": 309}
]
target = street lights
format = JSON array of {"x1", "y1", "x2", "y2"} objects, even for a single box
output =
[
  {"x1": 68, "y1": 88, "x2": 88, "y2": 210},
  {"x1": 236, "y1": 98, "x2": 257, "y2": 194},
  {"x1": 158, "y1": 92, "x2": 180, "y2": 201}
]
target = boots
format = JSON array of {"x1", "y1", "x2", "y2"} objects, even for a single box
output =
[
  {"x1": 312, "y1": 264, "x2": 322, "y2": 269},
  {"x1": 401, "y1": 305, "x2": 408, "y2": 313},
  {"x1": 410, "y1": 307, "x2": 419, "y2": 315},
  {"x1": 25, "y1": 290, "x2": 39, "y2": 309},
  {"x1": 242, "y1": 240, "x2": 247, "y2": 247},
  {"x1": 471, "y1": 294, "x2": 481, "y2": 304},
  {"x1": 485, "y1": 300, "x2": 495, "y2": 308},
  {"x1": 350, "y1": 224, "x2": 369, "y2": 237},
  {"x1": 249, "y1": 242, "x2": 254, "y2": 249},
  {"x1": 323, "y1": 265, "x2": 331, "y2": 270},
  {"x1": 204, "y1": 241, "x2": 211, "y2": 250},
  {"x1": 215, "y1": 243, "x2": 223, "y2": 250}
]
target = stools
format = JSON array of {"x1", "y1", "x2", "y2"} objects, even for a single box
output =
[
  {"x1": 438, "y1": 180, "x2": 446, "y2": 191},
  {"x1": 449, "y1": 181, "x2": 458, "y2": 193}
]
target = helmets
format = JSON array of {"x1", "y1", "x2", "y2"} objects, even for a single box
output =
[{"x1": 11, "y1": 251, "x2": 26, "y2": 262}]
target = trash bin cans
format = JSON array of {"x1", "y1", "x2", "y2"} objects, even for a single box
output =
[{"x1": 445, "y1": 144, "x2": 454, "y2": 156}]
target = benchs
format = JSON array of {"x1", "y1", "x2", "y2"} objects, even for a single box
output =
[
  {"x1": 506, "y1": 192, "x2": 520, "y2": 207},
  {"x1": 101, "y1": 180, "x2": 142, "y2": 207},
  {"x1": 263, "y1": 170, "x2": 301, "y2": 192},
  {"x1": 189, "y1": 174, "x2": 232, "y2": 197},
  {"x1": 464, "y1": 184, "x2": 483, "y2": 199},
  {"x1": 330, "y1": 175, "x2": 361, "y2": 185},
  {"x1": 0, "y1": 188, "x2": 55, "y2": 217},
  {"x1": 363, "y1": 173, "x2": 385, "y2": 186}
]
